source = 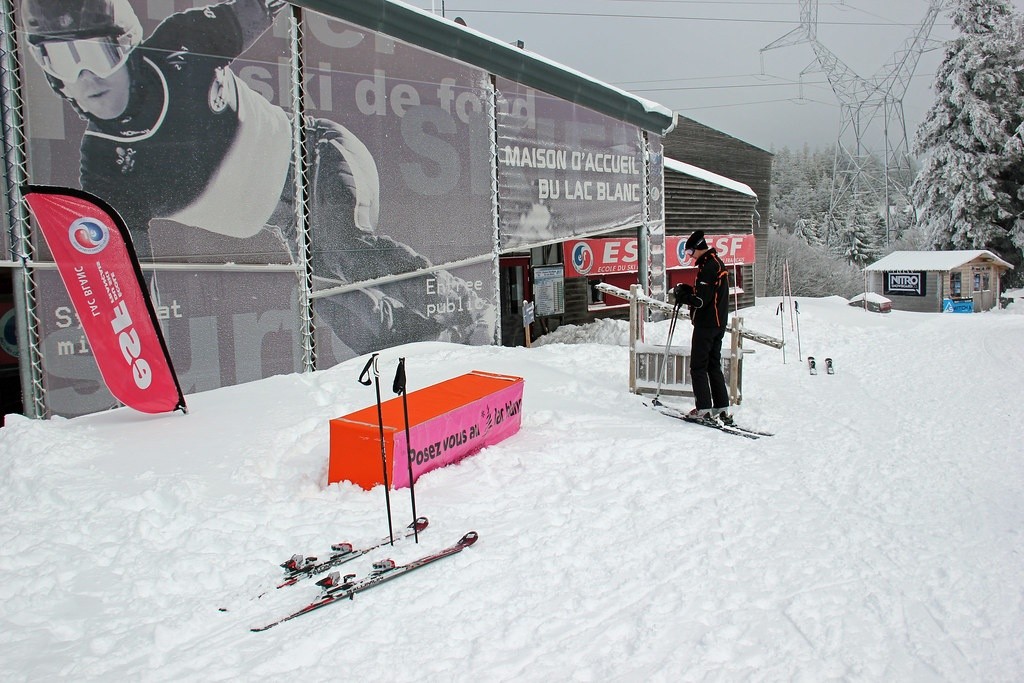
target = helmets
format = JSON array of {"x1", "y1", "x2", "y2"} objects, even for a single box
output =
[{"x1": 21, "y1": 0, "x2": 143, "y2": 99}]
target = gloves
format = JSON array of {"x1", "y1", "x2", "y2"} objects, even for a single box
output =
[
  {"x1": 676, "y1": 294, "x2": 698, "y2": 304},
  {"x1": 673, "y1": 284, "x2": 693, "y2": 294}
]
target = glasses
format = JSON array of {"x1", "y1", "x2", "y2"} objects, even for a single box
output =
[
  {"x1": 685, "y1": 249, "x2": 694, "y2": 257},
  {"x1": 37, "y1": 35, "x2": 127, "y2": 83}
]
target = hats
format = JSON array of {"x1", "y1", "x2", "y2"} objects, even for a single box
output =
[{"x1": 685, "y1": 230, "x2": 709, "y2": 250}]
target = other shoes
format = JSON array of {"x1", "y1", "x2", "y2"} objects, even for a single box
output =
[
  {"x1": 827, "y1": 361, "x2": 832, "y2": 368},
  {"x1": 810, "y1": 361, "x2": 815, "y2": 368}
]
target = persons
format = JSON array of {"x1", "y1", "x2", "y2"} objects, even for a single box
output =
[
  {"x1": 673, "y1": 229, "x2": 731, "y2": 424},
  {"x1": 19, "y1": 0, "x2": 495, "y2": 356}
]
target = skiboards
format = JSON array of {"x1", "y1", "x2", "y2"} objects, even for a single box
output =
[
  {"x1": 217, "y1": 518, "x2": 477, "y2": 634},
  {"x1": 641, "y1": 400, "x2": 775, "y2": 440}
]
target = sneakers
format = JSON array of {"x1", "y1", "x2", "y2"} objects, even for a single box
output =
[
  {"x1": 688, "y1": 408, "x2": 713, "y2": 418},
  {"x1": 711, "y1": 407, "x2": 730, "y2": 418},
  {"x1": 461, "y1": 305, "x2": 496, "y2": 347}
]
